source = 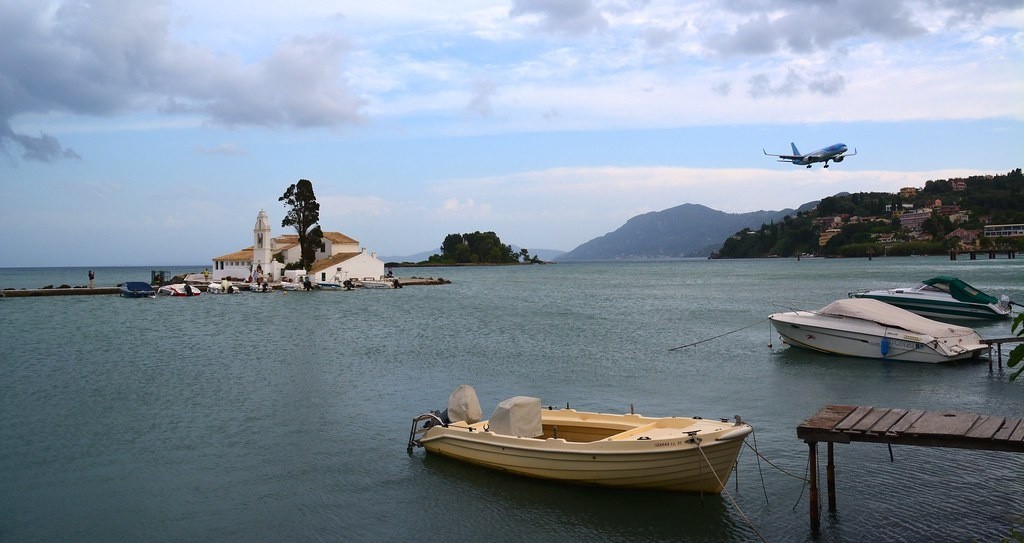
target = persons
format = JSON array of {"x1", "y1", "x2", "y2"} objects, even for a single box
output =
[
  {"x1": 253, "y1": 270, "x2": 264, "y2": 286},
  {"x1": 89, "y1": 271, "x2": 94, "y2": 288},
  {"x1": 155, "y1": 274, "x2": 161, "y2": 283},
  {"x1": 204, "y1": 268, "x2": 209, "y2": 281}
]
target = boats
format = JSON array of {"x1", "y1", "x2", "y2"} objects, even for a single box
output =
[
  {"x1": 405, "y1": 384, "x2": 754, "y2": 496},
  {"x1": 116, "y1": 281, "x2": 156, "y2": 298},
  {"x1": 315, "y1": 270, "x2": 356, "y2": 291},
  {"x1": 280, "y1": 274, "x2": 313, "y2": 292},
  {"x1": 206, "y1": 278, "x2": 239, "y2": 295},
  {"x1": 766, "y1": 295, "x2": 994, "y2": 365},
  {"x1": 846, "y1": 274, "x2": 1014, "y2": 321},
  {"x1": 361, "y1": 266, "x2": 403, "y2": 290},
  {"x1": 249, "y1": 282, "x2": 273, "y2": 294},
  {"x1": 157, "y1": 283, "x2": 202, "y2": 297}
]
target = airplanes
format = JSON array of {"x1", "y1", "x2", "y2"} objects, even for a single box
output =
[{"x1": 763, "y1": 142, "x2": 858, "y2": 169}]
